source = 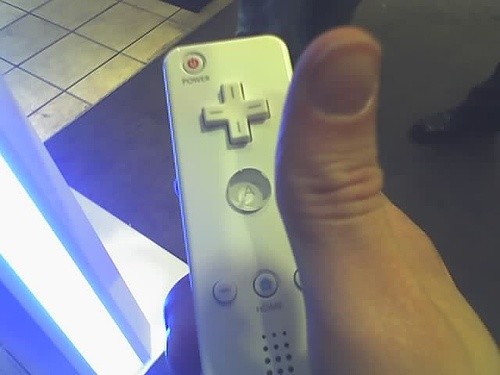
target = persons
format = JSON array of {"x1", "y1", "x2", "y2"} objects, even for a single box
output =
[{"x1": 161, "y1": 26, "x2": 500, "y2": 375}]
